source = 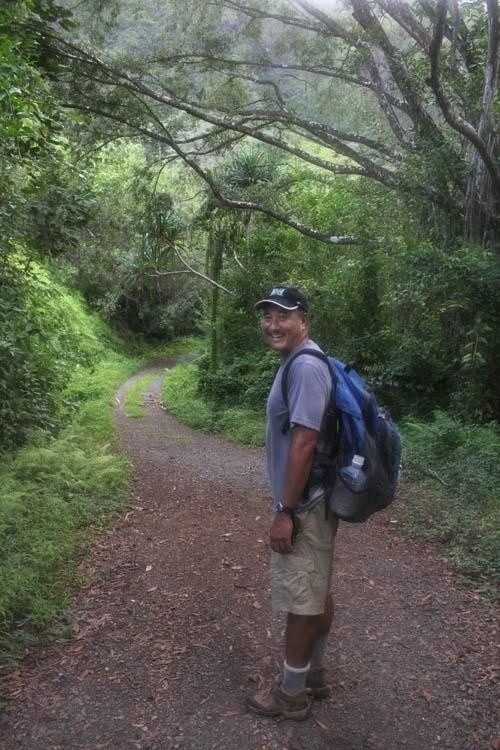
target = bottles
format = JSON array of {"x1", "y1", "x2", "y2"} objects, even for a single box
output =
[{"x1": 328, "y1": 454, "x2": 370, "y2": 519}]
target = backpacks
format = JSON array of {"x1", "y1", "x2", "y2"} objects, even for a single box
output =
[{"x1": 281, "y1": 349, "x2": 402, "y2": 524}]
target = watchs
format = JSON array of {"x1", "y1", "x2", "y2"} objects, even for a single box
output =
[{"x1": 274, "y1": 500, "x2": 297, "y2": 515}]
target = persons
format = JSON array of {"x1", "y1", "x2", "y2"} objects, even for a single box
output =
[{"x1": 242, "y1": 284, "x2": 338, "y2": 723}]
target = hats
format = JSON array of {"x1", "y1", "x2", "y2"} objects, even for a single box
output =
[{"x1": 255, "y1": 286, "x2": 308, "y2": 310}]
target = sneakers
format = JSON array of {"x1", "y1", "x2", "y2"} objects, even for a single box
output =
[
  {"x1": 246, "y1": 683, "x2": 313, "y2": 721},
  {"x1": 307, "y1": 668, "x2": 330, "y2": 697}
]
